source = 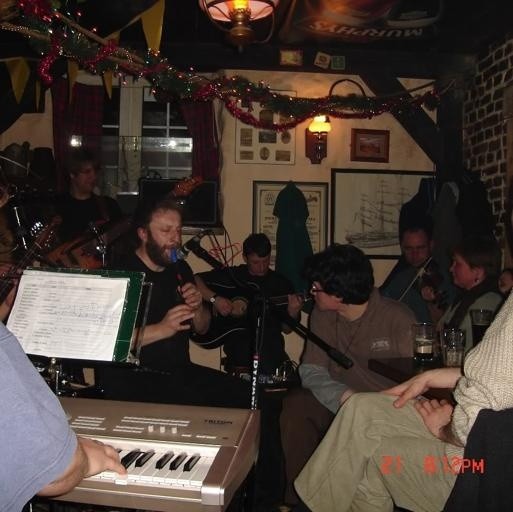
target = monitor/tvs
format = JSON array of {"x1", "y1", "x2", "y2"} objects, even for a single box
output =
[{"x1": 138, "y1": 178, "x2": 217, "y2": 226}]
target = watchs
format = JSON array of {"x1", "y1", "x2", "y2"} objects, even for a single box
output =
[{"x1": 208, "y1": 294, "x2": 217, "y2": 304}]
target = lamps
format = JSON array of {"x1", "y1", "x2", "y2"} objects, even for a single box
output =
[{"x1": 198, "y1": 0, "x2": 279, "y2": 54}]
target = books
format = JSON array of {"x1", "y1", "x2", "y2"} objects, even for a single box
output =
[
  {"x1": 22, "y1": 263, "x2": 146, "y2": 367},
  {"x1": 4, "y1": 268, "x2": 131, "y2": 363}
]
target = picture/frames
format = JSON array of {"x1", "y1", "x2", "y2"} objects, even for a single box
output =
[
  {"x1": 253, "y1": 167, "x2": 437, "y2": 272},
  {"x1": 350, "y1": 128, "x2": 389, "y2": 163}
]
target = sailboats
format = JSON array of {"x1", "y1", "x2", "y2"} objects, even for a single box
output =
[{"x1": 345, "y1": 180, "x2": 410, "y2": 249}]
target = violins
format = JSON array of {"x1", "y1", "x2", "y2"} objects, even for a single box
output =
[{"x1": 410, "y1": 260, "x2": 452, "y2": 307}]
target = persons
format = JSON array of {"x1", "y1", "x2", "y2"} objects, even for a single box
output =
[
  {"x1": 265, "y1": 242, "x2": 426, "y2": 512},
  {"x1": 107, "y1": 195, "x2": 269, "y2": 424},
  {"x1": 420, "y1": 229, "x2": 506, "y2": 351},
  {"x1": 273, "y1": 176, "x2": 512, "y2": 511},
  {"x1": 375, "y1": 210, "x2": 455, "y2": 326},
  {"x1": 27, "y1": 146, "x2": 134, "y2": 272},
  {"x1": 192, "y1": 231, "x2": 303, "y2": 379},
  {"x1": 0, "y1": 316, "x2": 128, "y2": 512}
]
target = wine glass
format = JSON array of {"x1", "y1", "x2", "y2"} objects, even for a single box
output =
[{"x1": 469, "y1": 308, "x2": 493, "y2": 339}]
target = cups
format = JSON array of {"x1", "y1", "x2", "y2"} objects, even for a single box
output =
[
  {"x1": 412, "y1": 322, "x2": 434, "y2": 362},
  {"x1": 440, "y1": 328, "x2": 467, "y2": 367}
]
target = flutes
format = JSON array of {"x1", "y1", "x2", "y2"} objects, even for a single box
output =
[{"x1": 170, "y1": 249, "x2": 200, "y2": 337}]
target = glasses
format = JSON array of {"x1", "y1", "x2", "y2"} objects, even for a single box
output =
[{"x1": 310, "y1": 284, "x2": 324, "y2": 296}]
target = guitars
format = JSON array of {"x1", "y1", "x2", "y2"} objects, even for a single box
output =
[
  {"x1": 46, "y1": 175, "x2": 202, "y2": 269},
  {"x1": 189, "y1": 284, "x2": 314, "y2": 350}
]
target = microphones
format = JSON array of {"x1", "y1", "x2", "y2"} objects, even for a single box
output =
[{"x1": 176, "y1": 229, "x2": 211, "y2": 261}]
